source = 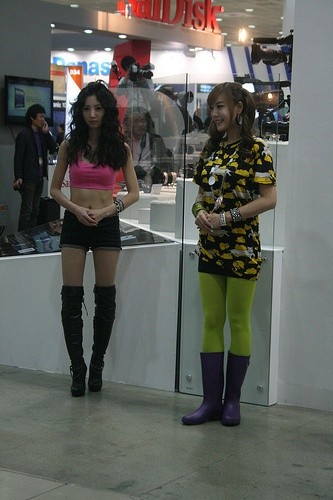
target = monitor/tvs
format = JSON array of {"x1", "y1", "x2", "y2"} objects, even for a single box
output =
[{"x1": 4, "y1": 76, "x2": 53, "y2": 127}]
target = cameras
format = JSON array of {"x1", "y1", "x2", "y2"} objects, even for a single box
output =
[{"x1": 140, "y1": 68, "x2": 153, "y2": 80}]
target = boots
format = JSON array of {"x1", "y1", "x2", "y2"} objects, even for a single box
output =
[
  {"x1": 182, "y1": 351, "x2": 224, "y2": 424},
  {"x1": 89, "y1": 284, "x2": 116, "y2": 391},
  {"x1": 221, "y1": 351, "x2": 251, "y2": 425},
  {"x1": 60, "y1": 285, "x2": 88, "y2": 396}
]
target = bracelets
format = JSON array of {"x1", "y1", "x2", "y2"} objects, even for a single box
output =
[
  {"x1": 114, "y1": 199, "x2": 125, "y2": 213},
  {"x1": 230, "y1": 207, "x2": 242, "y2": 222},
  {"x1": 219, "y1": 212, "x2": 228, "y2": 226}
]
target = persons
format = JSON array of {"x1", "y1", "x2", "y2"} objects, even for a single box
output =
[
  {"x1": 56, "y1": 123, "x2": 65, "y2": 145},
  {"x1": 12, "y1": 103, "x2": 58, "y2": 232},
  {"x1": 123, "y1": 108, "x2": 180, "y2": 185},
  {"x1": 181, "y1": 81, "x2": 277, "y2": 425},
  {"x1": 50, "y1": 82, "x2": 139, "y2": 396},
  {"x1": 116, "y1": 56, "x2": 156, "y2": 109}
]
